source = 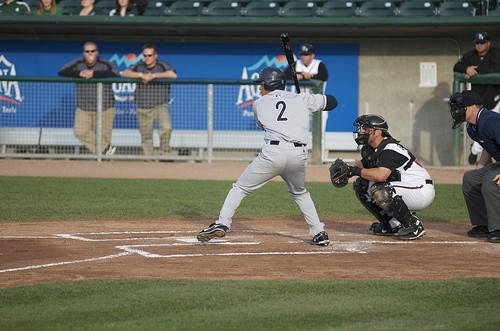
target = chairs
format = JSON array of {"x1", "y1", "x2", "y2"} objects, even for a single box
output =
[{"x1": 0, "y1": 0, "x2": 500, "y2": 18}]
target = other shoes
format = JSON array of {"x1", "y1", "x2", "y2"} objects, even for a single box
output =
[
  {"x1": 105, "y1": 145, "x2": 117, "y2": 156},
  {"x1": 469, "y1": 145, "x2": 477, "y2": 164}
]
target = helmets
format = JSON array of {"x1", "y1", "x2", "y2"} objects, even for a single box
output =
[
  {"x1": 362, "y1": 115, "x2": 392, "y2": 138},
  {"x1": 474, "y1": 32, "x2": 489, "y2": 44},
  {"x1": 252, "y1": 67, "x2": 286, "y2": 90}
]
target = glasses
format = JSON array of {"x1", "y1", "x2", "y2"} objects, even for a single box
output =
[
  {"x1": 84, "y1": 50, "x2": 97, "y2": 53},
  {"x1": 144, "y1": 54, "x2": 155, "y2": 57}
]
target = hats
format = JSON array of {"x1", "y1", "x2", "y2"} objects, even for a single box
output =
[
  {"x1": 299, "y1": 43, "x2": 313, "y2": 55},
  {"x1": 460, "y1": 90, "x2": 480, "y2": 105}
]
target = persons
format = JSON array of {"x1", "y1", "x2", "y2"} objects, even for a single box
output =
[
  {"x1": 450, "y1": 90, "x2": 500, "y2": 243},
  {"x1": 453, "y1": 31, "x2": 500, "y2": 165},
  {"x1": 0, "y1": 0, "x2": 137, "y2": 16},
  {"x1": 196, "y1": 67, "x2": 338, "y2": 246},
  {"x1": 57, "y1": 42, "x2": 120, "y2": 161},
  {"x1": 120, "y1": 43, "x2": 178, "y2": 162},
  {"x1": 283, "y1": 43, "x2": 327, "y2": 160},
  {"x1": 330, "y1": 114, "x2": 435, "y2": 240}
]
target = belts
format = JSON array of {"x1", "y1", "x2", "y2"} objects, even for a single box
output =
[{"x1": 270, "y1": 140, "x2": 303, "y2": 147}]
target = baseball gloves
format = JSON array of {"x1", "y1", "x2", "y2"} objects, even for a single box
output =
[{"x1": 330, "y1": 158, "x2": 350, "y2": 189}]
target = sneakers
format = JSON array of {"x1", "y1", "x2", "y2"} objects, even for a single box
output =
[
  {"x1": 467, "y1": 226, "x2": 490, "y2": 237},
  {"x1": 197, "y1": 223, "x2": 225, "y2": 243},
  {"x1": 372, "y1": 225, "x2": 405, "y2": 236},
  {"x1": 488, "y1": 231, "x2": 500, "y2": 243},
  {"x1": 399, "y1": 223, "x2": 425, "y2": 240},
  {"x1": 312, "y1": 231, "x2": 329, "y2": 246}
]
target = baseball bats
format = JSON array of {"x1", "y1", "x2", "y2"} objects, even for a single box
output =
[{"x1": 280, "y1": 32, "x2": 301, "y2": 94}]
